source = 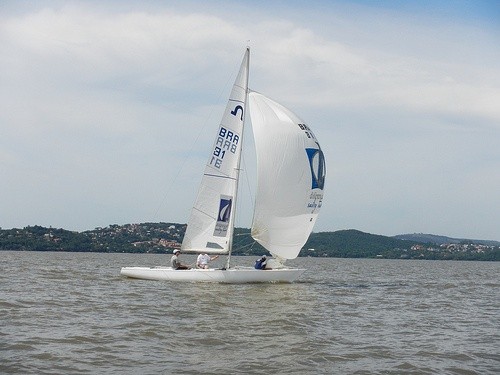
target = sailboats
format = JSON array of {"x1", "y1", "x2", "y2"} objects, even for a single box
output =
[{"x1": 119, "y1": 46, "x2": 325, "y2": 283}]
